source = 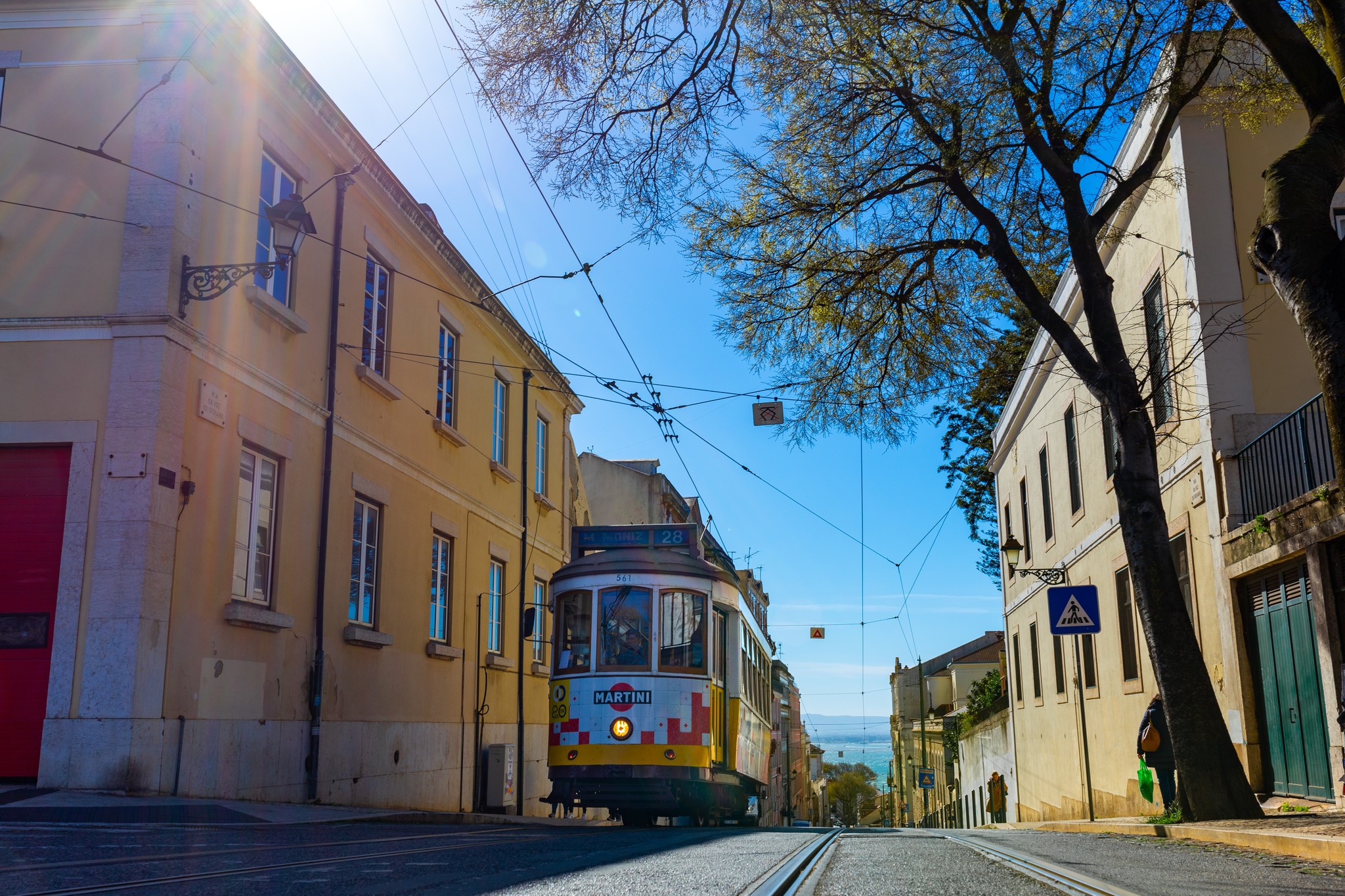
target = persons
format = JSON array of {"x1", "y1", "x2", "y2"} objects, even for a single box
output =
[
  {"x1": 611, "y1": 630, "x2": 681, "y2": 666},
  {"x1": 566, "y1": 807, "x2": 587, "y2": 820},
  {"x1": 1136, "y1": 693, "x2": 1176, "y2": 810},
  {"x1": 946, "y1": 805, "x2": 954, "y2": 828},
  {"x1": 986, "y1": 770, "x2": 1008, "y2": 823},
  {"x1": 606, "y1": 809, "x2": 621, "y2": 822},
  {"x1": 548, "y1": 783, "x2": 568, "y2": 818}
]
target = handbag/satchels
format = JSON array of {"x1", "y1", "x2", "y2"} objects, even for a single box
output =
[
  {"x1": 1137, "y1": 756, "x2": 1154, "y2": 803},
  {"x1": 1141, "y1": 710, "x2": 1160, "y2": 752}
]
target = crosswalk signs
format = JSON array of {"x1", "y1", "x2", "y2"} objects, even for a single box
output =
[
  {"x1": 884, "y1": 819, "x2": 890, "y2": 826},
  {"x1": 1047, "y1": 584, "x2": 1101, "y2": 636}
]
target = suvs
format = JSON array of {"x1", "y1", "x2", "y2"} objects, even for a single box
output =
[{"x1": 792, "y1": 819, "x2": 812, "y2": 827}]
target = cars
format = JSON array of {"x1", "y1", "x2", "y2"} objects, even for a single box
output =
[{"x1": 831, "y1": 817, "x2": 844, "y2": 828}]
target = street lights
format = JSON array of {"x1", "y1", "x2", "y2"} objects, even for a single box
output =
[
  {"x1": 829, "y1": 803, "x2": 832, "y2": 827},
  {"x1": 907, "y1": 754, "x2": 929, "y2": 828},
  {"x1": 885, "y1": 799, "x2": 889, "y2": 819},
  {"x1": 821, "y1": 784, "x2": 826, "y2": 827},
  {"x1": 893, "y1": 784, "x2": 905, "y2": 827},
  {"x1": 787, "y1": 769, "x2": 798, "y2": 827}
]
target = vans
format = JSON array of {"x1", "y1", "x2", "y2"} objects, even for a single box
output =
[{"x1": 745, "y1": 796, "x2": 761, "y2": 826}]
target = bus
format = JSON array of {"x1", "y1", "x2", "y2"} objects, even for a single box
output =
[{"x1": 523, "y1": 514, "x2": 776, "y2": 831}]
